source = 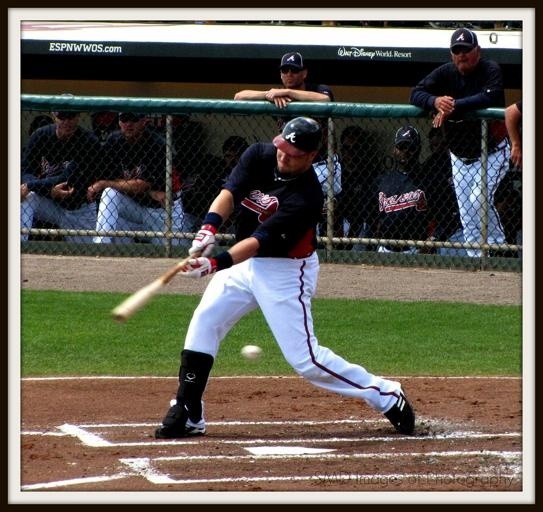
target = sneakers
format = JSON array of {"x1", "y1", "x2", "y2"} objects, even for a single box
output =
[
  {"x1": 384, "y1": 382, "x2": 414, "y2": 435},
  {"x1": 155, "y1": 399, "x2": 206, "y2": 438}
]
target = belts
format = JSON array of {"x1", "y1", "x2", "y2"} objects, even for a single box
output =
[
  {"x1": 460, "y1": 144, "x2": 508, "y2": 165},
  {"x1": 170, "y1": 190, "x2": 184, "y2": 201}
]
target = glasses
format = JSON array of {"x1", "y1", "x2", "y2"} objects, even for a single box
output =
[
  {"x1": 282, "y1": 67, "x2": 301, "y2": 74},
  {"x1": 451, "y1": 46, "x2": 471, "y2": 54},
  {"x1": 121, "y1": 117, "x2": 138, "y2": 122},
  {"x1": 56, "y1": 111, "x2": 76, "y2": 120}
]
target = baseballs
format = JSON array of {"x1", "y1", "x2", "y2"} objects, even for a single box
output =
[{"x1": 243, "y1": 346, "x2": 262, "y2": 360}]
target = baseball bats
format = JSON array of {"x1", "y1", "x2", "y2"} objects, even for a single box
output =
[{"x1": 111, "y1": 250, "x2": 200, "y2": 321}]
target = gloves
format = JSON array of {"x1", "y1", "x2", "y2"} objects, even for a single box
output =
[{"x1": 177, "y1": 224, "x2": 217, "y2": 279}]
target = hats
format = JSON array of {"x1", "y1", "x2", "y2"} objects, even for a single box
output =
[
  {"x1": 451, "y1": 28, "x2": 477, "y2": 48},
  {"x1": 394, "y1": 126, "x2": 421, "y2": 144},
  {"x1": 280, "y1": 52, "x2": 304, "y2": 68}
]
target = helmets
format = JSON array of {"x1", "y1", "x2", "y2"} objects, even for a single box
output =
[{"x1": 272, "y1": 116, "x2": 323, "y2": 157}]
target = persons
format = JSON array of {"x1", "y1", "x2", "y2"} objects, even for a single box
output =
[
  {"x1": 410, "y1": 27, "x2": 509, "y2": 257},
  {"x1": 22, "y1": 94, "x2": 521, "y2": 257},
  {"x1": 234, "y1": 51, "x2": 342, "y2": 235},
  {"x1": 154, "y1": 116, "x2": 414, "y2": 437}
]
